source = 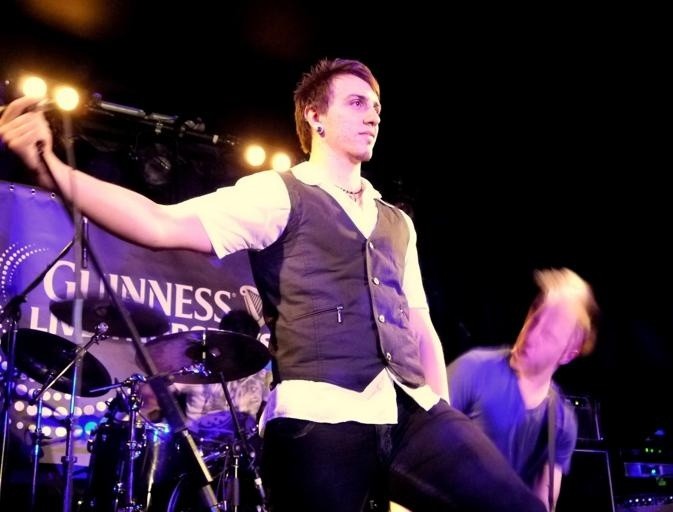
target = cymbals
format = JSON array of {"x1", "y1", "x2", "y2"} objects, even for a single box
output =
[
  {"x1": 0, "y1": 328, "x2": 110, "y2": 397},
  {"x1": 48, "y1": 300, "x2": 170, "y2": 337},
  {"x1": 135, "y1": 329, "x2": 271, "y2": 384}
]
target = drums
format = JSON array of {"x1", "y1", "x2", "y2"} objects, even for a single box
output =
[
  {"x1": 189, "y1": 409, "x2": 261, "y2": 464},
  {"x1": 81, "y1": 424, "x2": 176, "y2": 512},
  {"x1": 149, "y1": 445, "x2": 268, "y2": 512}
]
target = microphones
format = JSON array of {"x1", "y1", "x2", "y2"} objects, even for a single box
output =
[{"x1": 94, "y1": 322, "x2": 109, "y2": 336}]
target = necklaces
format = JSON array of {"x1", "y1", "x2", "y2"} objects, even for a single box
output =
[{"x1": 335, "y1": 183, "x2": 362, "y2": 206}]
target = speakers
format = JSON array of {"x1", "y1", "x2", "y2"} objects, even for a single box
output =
[{"x1": 556, "y1": 394, "x2": 673, "y2": 512}]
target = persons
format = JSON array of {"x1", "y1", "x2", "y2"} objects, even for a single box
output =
[
  {"x1": 133, "y1": 305, "x2": 271, "y2": 511},
  {"x1": 0, "y1": 56, "x2": 548, "y2": 512},
  {"x1": 444, "y1": 264, "x2": 599, "y2": 512}
]
